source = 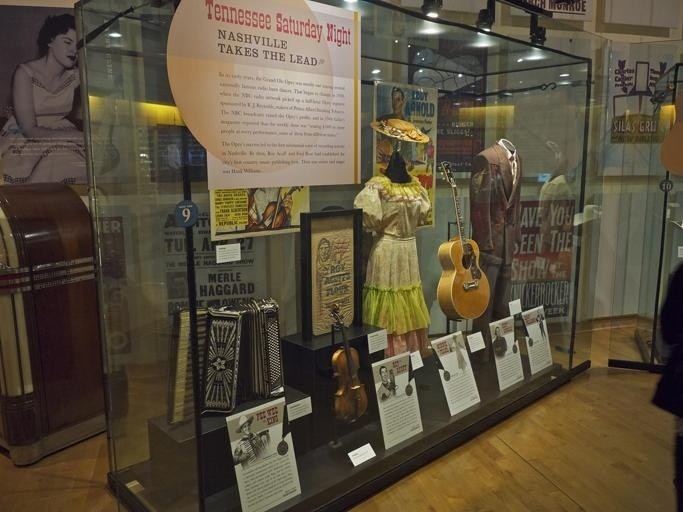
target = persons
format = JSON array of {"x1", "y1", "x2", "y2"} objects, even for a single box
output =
[
  {"x1": 473, "y1": 138, "x2": 521, "y2": 329},
  {"x1": 247, "y1": 188, "x2": 293, "y2": 230},
  {"x1": 535, "y1": 310, "x2": 547, "y2": 340},
  {"x1": 318, "y1": 237, "x2": 332, "y2": 265},
  {"x1": 492, "y1": 327, "x2": 508, "y2": 359},
  {"x1": 358, "y1": 154, "x2": 427, "y2": 361},
  {"x1": 451, "y1": 337, "x2": 467, "y2": 373},
  {"x1": 374, "y1": 88, "x2": 412, "y2": 155},
  {"x1": 375, "y1": 367, "x2": 396, "y2": 401},
  {"x1": 1, "y1": 16, "x2": 87, "y2": 179},
  {"x1": 233, "y1": 414, "x2": 261, "y2": 469}
]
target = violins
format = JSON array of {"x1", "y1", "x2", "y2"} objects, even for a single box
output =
[{"x1": 329, "y1": 302, "x2": 368, "y2": 425}]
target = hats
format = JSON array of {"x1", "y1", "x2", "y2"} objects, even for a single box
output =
[
  {"x1": 235, "y1": 415, "x2": 253, "y2": 433},
  {"x1": 370, "y1": 119, "x2": 431, "y2": 144}
]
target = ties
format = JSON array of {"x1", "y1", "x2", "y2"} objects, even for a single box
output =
[{"x1": 510, "y1": 156, "x2": 516, "y2": 186}]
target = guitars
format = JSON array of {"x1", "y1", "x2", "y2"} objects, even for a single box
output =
[{"x1": 438, "y1": 160, "x2": 491, "y2": 321}]
